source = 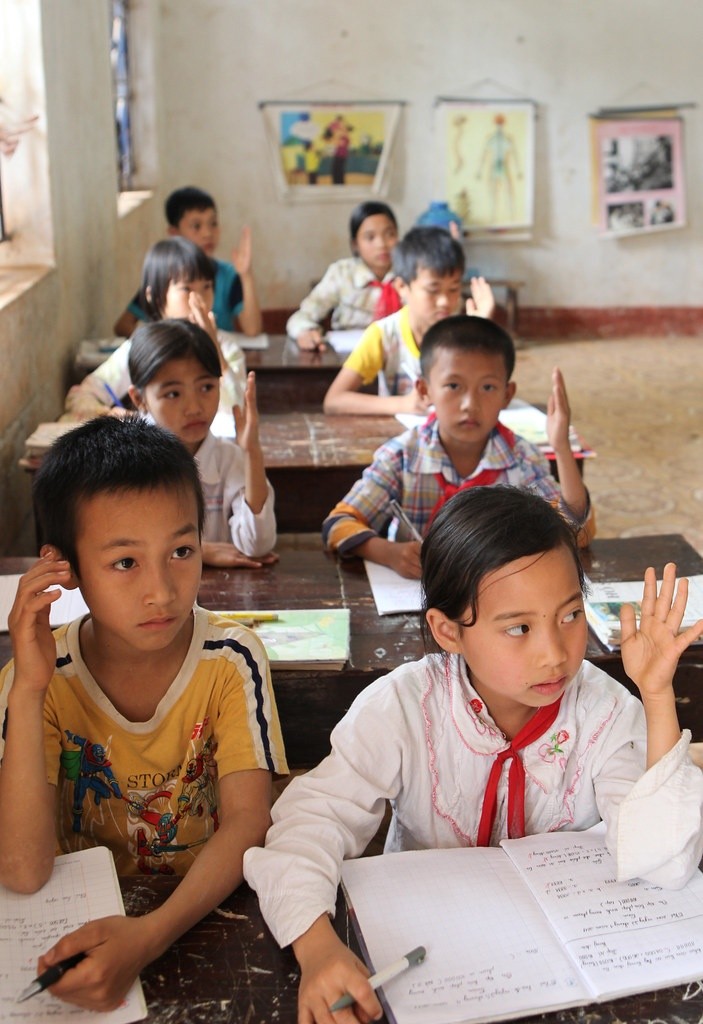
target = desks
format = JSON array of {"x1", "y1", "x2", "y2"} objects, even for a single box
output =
[
  {"x1": 0, "y1": 854, "x2": 703, "y2": 1024},
  {"x1": 0, "y1": 529, "x2": 703, "y2": 769},
  {"x1": 75, "y1": 331, "x2": 379, "y2": 413},
  {"x1": 16, "y1": 402, "x2": 598, "y2": 556}
]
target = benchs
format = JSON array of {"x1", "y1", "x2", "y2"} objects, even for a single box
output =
[{"x1": 311, "y1": 278, "x2": 526, "y2": 350}]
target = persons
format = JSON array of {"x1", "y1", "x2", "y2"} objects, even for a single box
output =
[
  {"x1": 319, "y1": 315, "x2": 598, "y2": 580},
  {"x1": 240, "y1": 483, "x2": 702, "y2": 1024},
  {"x1": 322, "y1": 226, "x2": 495, "y2": 417},
  {"x1": 284, "y1": 201, "x2": 461, "y2": 353},
  {"x1": 113, "y1": 185, "x2": 262, "y2": 343},
  {"x1": 63, "y1": 236, "x2": 249, "y2": 421},
  {"x1": 1, "y1": 414, "x2": 290, "y2": 1014},
  {"x1": 114, "y1": 318, "x2": 282, "y2": 568}
]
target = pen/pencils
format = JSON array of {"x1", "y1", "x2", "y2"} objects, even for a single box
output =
[
  {"x1": 390, "y1": 499, "x2": 428, "y2": 545},
  {"x1": 326, "y1": 944, "x2": 427, "y2": 1014},
  {"x1": 311, "y1": 314, "x2": 332, "y2": 356},
  {"x1": 98, "y1": 378, "x2": 126, "y2": 411},
  {"x1": 18, "y1": 950, "x2": 89, "y2": 1004},
  {"x1": 220, "y1": 611, "x2": 279, "y2": 626},
  {"x1": 400, "y1": 362, "x2": 419, "y2": 383}
]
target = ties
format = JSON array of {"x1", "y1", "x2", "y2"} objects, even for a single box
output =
[
  {"x1": 367, "y1": 280, "x2": 402, "y2": 322},
  {"x1": 422, "y1": 413, "x2": 514, "y2": 537},
  {"x1": 476, "y1": 687, "x2": 565, "y2": 848}
]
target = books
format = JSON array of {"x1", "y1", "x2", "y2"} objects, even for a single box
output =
[
  {"x1": 362, "y1": 556, "x2": 427, "y2": 617},
  {"x1": 581, "y1": 573, "x2": 701, "y2": 653},
  {"x1": 74, "y1": 328, "x2": 366, "y2": 366},
  {"x1": 1, "y1": 574, "x2": 91, "y2": 631},
  {"x1": 208, "y1": 608, "x2": 352, "y2": 671},
  {"x1": 0, "y1": 847, "x2": 149, "y2": 1024},
  {"x1": 338, "y1": 817, "x2": 702, "y2": 1024},
  {"x1": 17, "y1": 398, "x2": 586, "y2": 471}
]
